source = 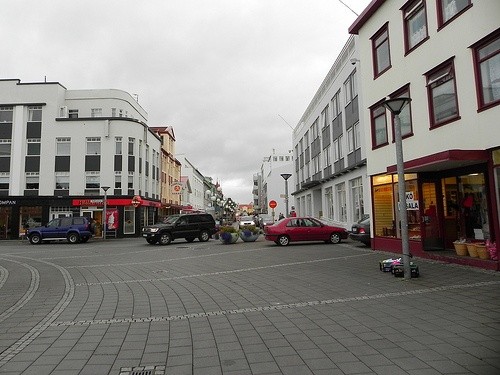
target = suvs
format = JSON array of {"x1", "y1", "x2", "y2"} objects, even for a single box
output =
[
  {"x1": 141, "y1": 213, "x2": 217, "y2": 246},
  {"x1": 25, "y1": 216, "x2": 97, "y2": 244}
]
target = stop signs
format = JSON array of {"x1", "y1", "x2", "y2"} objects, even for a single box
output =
[{"x1": 269, "y1": 201, "x2": 277, "y2": 209}]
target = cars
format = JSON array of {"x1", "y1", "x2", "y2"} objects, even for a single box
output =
[
  {"x1": 260, "y1": 217, "x2": 276, "y2": 230},
  {"x1": 264, "y1": 216, "x2": 349, "y2": 246},
  {"x1": 349, "y1": 216, "x2": 371, "y2": 247},
  {"x1": 239, "y1": 216, "x2": 257, "y2": 229}
]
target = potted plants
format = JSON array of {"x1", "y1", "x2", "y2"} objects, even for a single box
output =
[
  {"x1": 218, "y1": 225, "x2": 239, "y2": 243},
  {"x1": 240, "y1": 225, "x2": 260, "y2": 242}
]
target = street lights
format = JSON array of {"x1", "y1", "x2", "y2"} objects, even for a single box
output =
[
  {"x1": 381, "y1": 97, "x2": 414, "y2": 281},
  {"x1": 100, "y1": 186, "x2": 110, "y2": 240},
  {"x1": 280, "y1": 173, "x2": 292, "y2": 218}
]
